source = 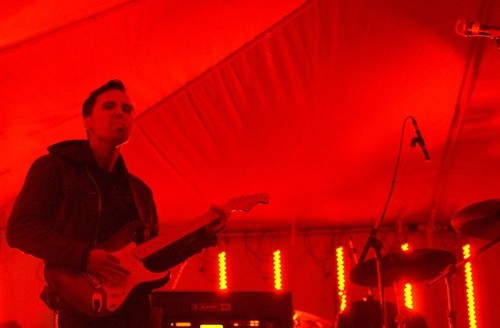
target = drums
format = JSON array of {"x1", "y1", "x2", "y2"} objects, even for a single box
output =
[{"x1": 338, "y1": 300, "x2": 429, "y2": 328}]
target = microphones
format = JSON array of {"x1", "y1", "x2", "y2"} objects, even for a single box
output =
[
  {"x1": 455, "y1": 20, "x2": 500, "y2": 39},
  {"x1": 409, "y1": 118, "x2": 430, "y2": 162}
]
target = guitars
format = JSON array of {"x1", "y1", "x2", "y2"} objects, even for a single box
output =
[{"x1": 41, "y1": 192, "x2": 272, "y2": 321}]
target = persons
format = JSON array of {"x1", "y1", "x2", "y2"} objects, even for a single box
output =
[{"x1": 6, "y1": 80, "x2": 225, "y2": 327}]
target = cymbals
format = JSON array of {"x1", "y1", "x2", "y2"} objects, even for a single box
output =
[
  {"x1": 451, "y1": 196, "x2": 500, "y2": 243},
  {"x1": 348, "y1": 247, "x2": 458, "y2": 288}
]
